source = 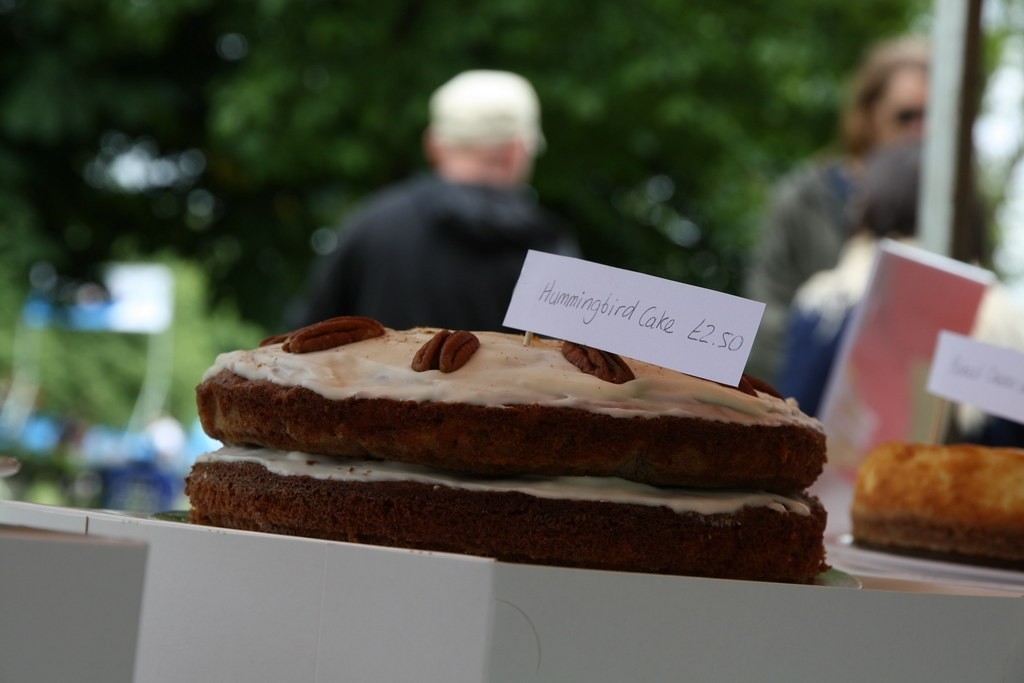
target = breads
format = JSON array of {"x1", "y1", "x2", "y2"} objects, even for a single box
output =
[{"x1": 847, "y1": 439, "x2": 1024, "y2": 571}]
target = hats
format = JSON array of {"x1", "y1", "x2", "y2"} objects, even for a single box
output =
[{"x1": 428, "y1": 70, "x2": 541, "y2": 139}]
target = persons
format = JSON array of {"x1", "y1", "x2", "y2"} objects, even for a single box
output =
[
  {"x1": 745, "y1": 37, "x2": 1006, "y2": 427},
  {"x1": 267, "y1": 70, "x2": 592, "y2": 341}
]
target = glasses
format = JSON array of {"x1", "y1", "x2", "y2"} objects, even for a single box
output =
[{"x1": 897, "y1": 108, "x2": 920, "y2": 124}]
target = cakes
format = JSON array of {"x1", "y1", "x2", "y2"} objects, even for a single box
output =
[{"x1": 183, "y1": 313, "x2": 829, "y2": 586}]
